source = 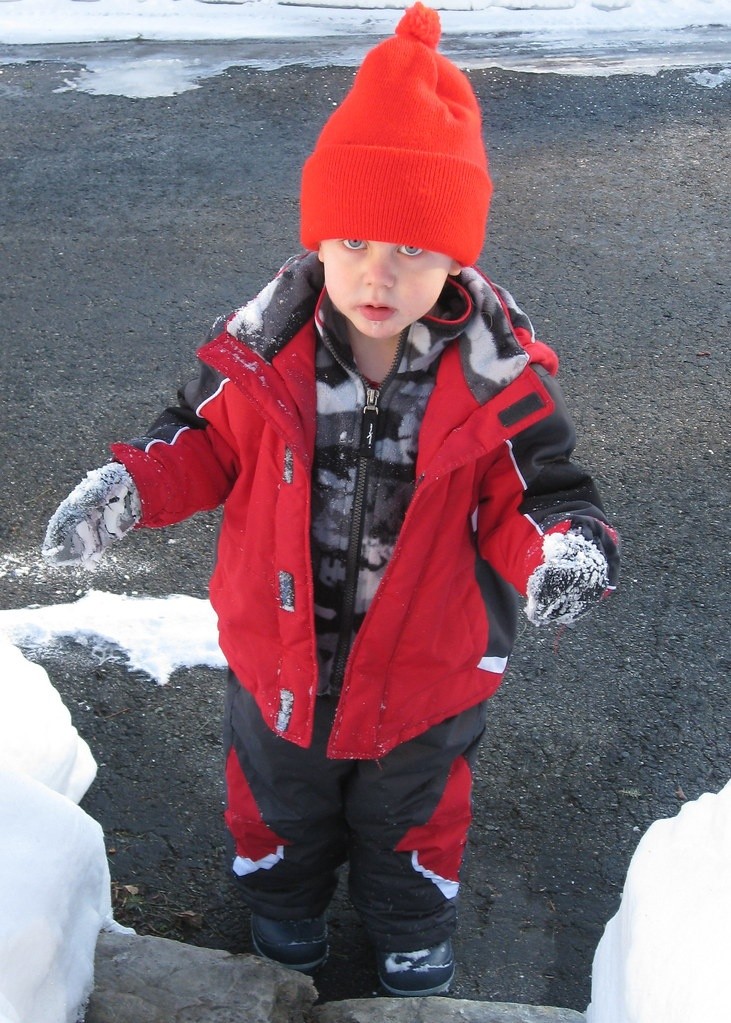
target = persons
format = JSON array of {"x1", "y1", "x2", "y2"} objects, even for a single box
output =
[{"x1": 45, "y1": 3, "x2": 621, "y2": 997}]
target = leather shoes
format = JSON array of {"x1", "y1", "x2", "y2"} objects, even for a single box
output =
[
  {"x1": 251, "y1": 911, "x2": 329, "y2": 969},
  {"x1": 376, "y1": 935, "x2": 454, "y2": 996}
]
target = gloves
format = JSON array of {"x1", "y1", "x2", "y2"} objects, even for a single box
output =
[
  {"x1": 526, "y1": 529, "x2": 610, "y2": 625},
  {"x1": 41, "y1": 462, "x2": 142, "y2": 570}
]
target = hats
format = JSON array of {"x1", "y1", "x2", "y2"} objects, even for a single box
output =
[{"x1": 299, "y1": 1, "x2": 493, "y2": 269}]
